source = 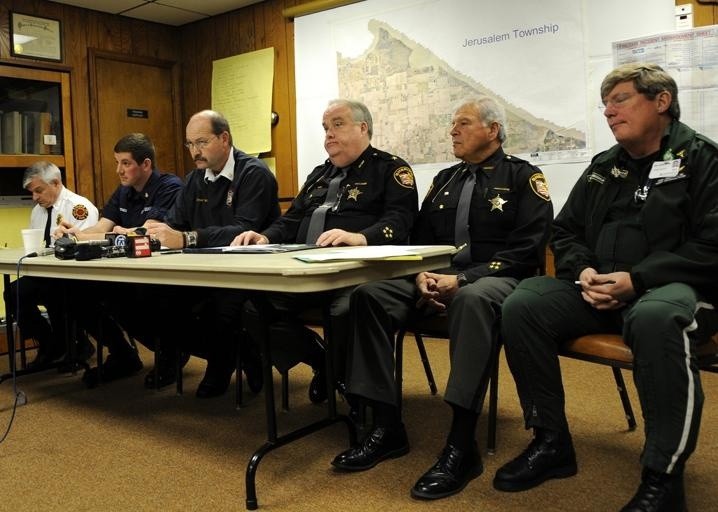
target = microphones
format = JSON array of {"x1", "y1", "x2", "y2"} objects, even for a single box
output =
[
  {"x1": 26, "y1": 249, "x2": 55, "y2": 257},
  {"x1": 73, "y1": 236, "x2": 161, "y2": 261},
  {"x1": 56, "y1": 232, "x2": 127, "y2": 257}
]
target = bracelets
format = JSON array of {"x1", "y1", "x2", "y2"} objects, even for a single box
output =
[{"x1": 181, "y1": 232, "x2": 187, "y2": 249}]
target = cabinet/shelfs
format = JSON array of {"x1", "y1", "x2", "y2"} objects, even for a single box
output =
[{"x1": 1, "y1": 63, "x2": 77, "y2": 356}]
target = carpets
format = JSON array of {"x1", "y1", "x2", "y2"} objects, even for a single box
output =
[{"x1": 1, "y1": 336, "x2": 718, "y2": 512}]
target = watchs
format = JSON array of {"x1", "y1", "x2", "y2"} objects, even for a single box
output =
[
  {"x1": 456, "y1": 273, "x2": 469, "y2": 288},
  {"x1": 184, "y1": 230, "x2": 197, "y2": 248}
]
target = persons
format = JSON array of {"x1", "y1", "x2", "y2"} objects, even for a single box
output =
[
  {"x1": 140, "y1": 112, "x2": 282, "y2": 397},
  {"x1": 54, "y1": 133, "x2": 193, "y2": 385},
  {"x1": 331, "y1": 100, "x2": 554, "y2": 500},
  {"x1": 4, "y1": 161, "x2": 101, "y2": 372},
  {"x1": 232, "y1": 100, "x2": 417, "y2": 403},
  {"x1": 497, "y1": 62, "x2": 718, "y2": 512}
]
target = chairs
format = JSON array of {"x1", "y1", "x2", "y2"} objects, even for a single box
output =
[
  {"x1": 559, "y1": 333, "x2": 718, "y2": 433},
  {"x1": 394, "y1": 248, "x2": 554, "y2": 456}
]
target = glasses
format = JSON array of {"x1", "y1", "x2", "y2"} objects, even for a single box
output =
[
  {"x1": 599, "y1": 90, "x2": 640, "y2": 112},
  {"x1": 185, "y1": 134, "x2": 217, "y2": 149},
  {"x1": 322, "y1": 121, "x2": 360, "y2": 132}
]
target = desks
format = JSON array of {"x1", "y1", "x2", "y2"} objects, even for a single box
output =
[{"x1": 0, "y1": 245, "x2": 455, "y2": 511}]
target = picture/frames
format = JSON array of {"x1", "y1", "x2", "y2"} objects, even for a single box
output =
[{"x1": 9, "y1": 9, "x2": 64, "y2": 63}]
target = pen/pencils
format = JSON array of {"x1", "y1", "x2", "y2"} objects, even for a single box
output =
[
  {"x1": 159, "y1": 250, "x2": 183, "y2": 255},
  {"x1": 573, "y1": 280, "x2": 616, "y2": 285}
]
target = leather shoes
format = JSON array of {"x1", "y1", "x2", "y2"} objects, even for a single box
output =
[
  {"x1": 410, "y1": 442, "x2": 483, "y2": 499},
  {"x1": 145, "y1": 351, "x2": 180, "y2": 388},
  {"x1": 332, "y1": 423, "x2": 411, "y2": 472},
  {"x1": 27, "y1": 337, "x2": 70, "y2": 372},
  {"x1": 82, "y1": 349, "x2": 143, "y2": 387},
  {"x1": 620, "y1": 482, "x2": 685, "y2": 512},
  {"x1": 196, "y1": 361, "x2": 236, "y2": 398},
  {"x1": 242, "y1": 360, "x2": 264, "y2": 395},
  {"x1": 57, "y1": 337, "x2": 95, "y2": 372},
  {"x1": 333, "y1": 377, "x2": 359, "y2": 412},
  {"x1": 309, "y1": 363, "x2": 329, "y2": 404},
  {"x1": 494, "y1": 430, "x2": 579, "y2": 492}
]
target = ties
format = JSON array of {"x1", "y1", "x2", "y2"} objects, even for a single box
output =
[
  {"x1": 42, "y1": 206, "x2": 52, "y2": 248},
  {"x1": 306, "y1": 167, "x2": 348, "y2": 245},
  {"x1": 453, "y1": 165, "x2": 477, "y2": 265}
]
